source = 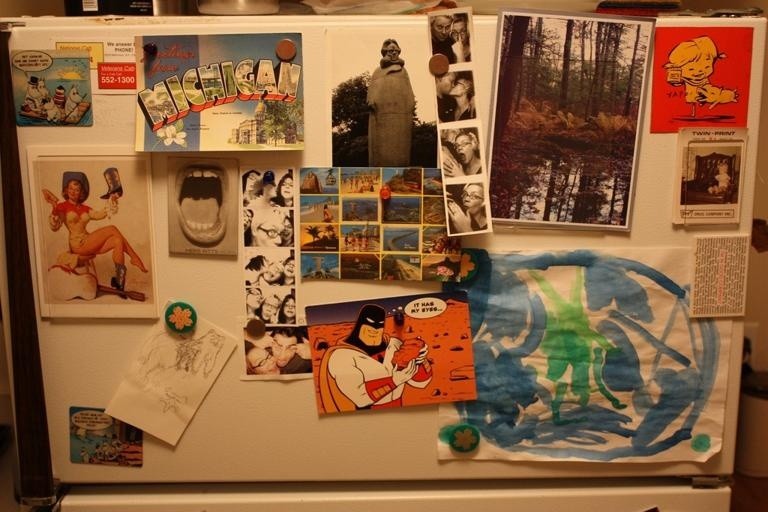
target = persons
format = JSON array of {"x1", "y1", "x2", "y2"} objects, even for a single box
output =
[
  {"x1": 445, "y1": 181, "x2": 488, "y2": 233},
  {"x1": 708, "y1": 163, "x2": 731, "y2": 196},
  {"x1": 251, "y1": 256, "x2": 284, "y2": 285},
  {"x1": 274, "y1": 174, "x2": 294, "y2": 208},
  {"x1": 256, "y1": 294, "x2": 282, "y2": 325},
  {"x1": 245, "y1": 287, "x2": 263, "y2": 324},
  {"x1": 444, "y1": 183, "x2": 482, "y2": 234},
  {"x1": 279, "y1": 294, "x2": 296, "y2": 324},
  {"x1": 441, "y1": 130, "x2": 462, "y2": 176},
  {"x1": 435, "y1": 70, "x2": 458, "y2": 122},
  {"x1": 450, "y1": 12, "x2": 472, "y2": 63},
  {"x1": 251, "y1": 210, "x2": 282, "y2": 246},
  {"x1": 271, "y1": 257, "x2": 295, "y2": 284},
  {"x1": 447, "y1": 75, "x2": 476, "y2": 121},
  {"x1": 367, "y1": 38, "x2": 419, "y2": 167},
  {"x1": 246, "y1": 172, "x2": 280, "y2": 206},
  {"x1": 42, "y1": 180, "x2": 147, "y2": 299},
  {"x1": 246, "y1": 246, "x2": 269, "y2": 286},
  {"x1": 242, "y1": 168, "x2": 260, "y2": 203},
  {"x1": 245, "y1": 340, "x2": 280, "y2": 375},
  {"x1": 430, "y1": 14, "x2": 457, "y2": 65},
  {"x1": 244, "y1": 209, "x2": 253, "y2": 245},
  {"x1": 443, "y1": 131, "x2": 483, "y2": 178},
  {"x1": 280, "y1": 209, "x2": 292, "y2": 246},
  {"x1": 265, "y1": 328, "x2": 312, "y2": 374}
]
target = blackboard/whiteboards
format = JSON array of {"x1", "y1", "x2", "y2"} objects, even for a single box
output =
[{"x1": 9, "y1": 20, "x2": 764, "y2": 496}]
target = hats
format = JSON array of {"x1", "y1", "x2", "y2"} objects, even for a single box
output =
[
  {"x1": 255, "y1": 171, "x2": 275, "y2": 197},
  {"x1": 62, "y1": 171, "x2": 90, "y2": 204}
]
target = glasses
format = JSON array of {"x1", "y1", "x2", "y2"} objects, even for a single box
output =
[
  {"x1": 285, "y1": 262, "x2": 295, "y2": 267},
  {"x1": 258, "y1": 227, "x2": 285, "y2": 238},
  {"x1": 452, "y1": 30, "x2": 467, "y2": 36},
  {"x1": 253, "y1": 351, "x2": 273, "y2": 367},
  {"x1": 247, "y1": 289, "x2": 264, "y2": 299},
  {"x1": 455, "y1": 81, "x2": 464, "y2": 85},
  {"x1": 263, "y1": 302, "x2": 280, "y2": 310},
  {"x1": 455, "y1": 142, "x2": 472, "y2": 148},
  {"x1": 462, "y1": 192, "x2": 483, "y2": 202}
]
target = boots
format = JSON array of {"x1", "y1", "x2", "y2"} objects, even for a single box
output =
[
  {"x1": 111, "y1": 264, "x2": 127, "y2": 300},
  {"x1": 100, "y1": 169, "x2": 123, "y2": 199}
]
